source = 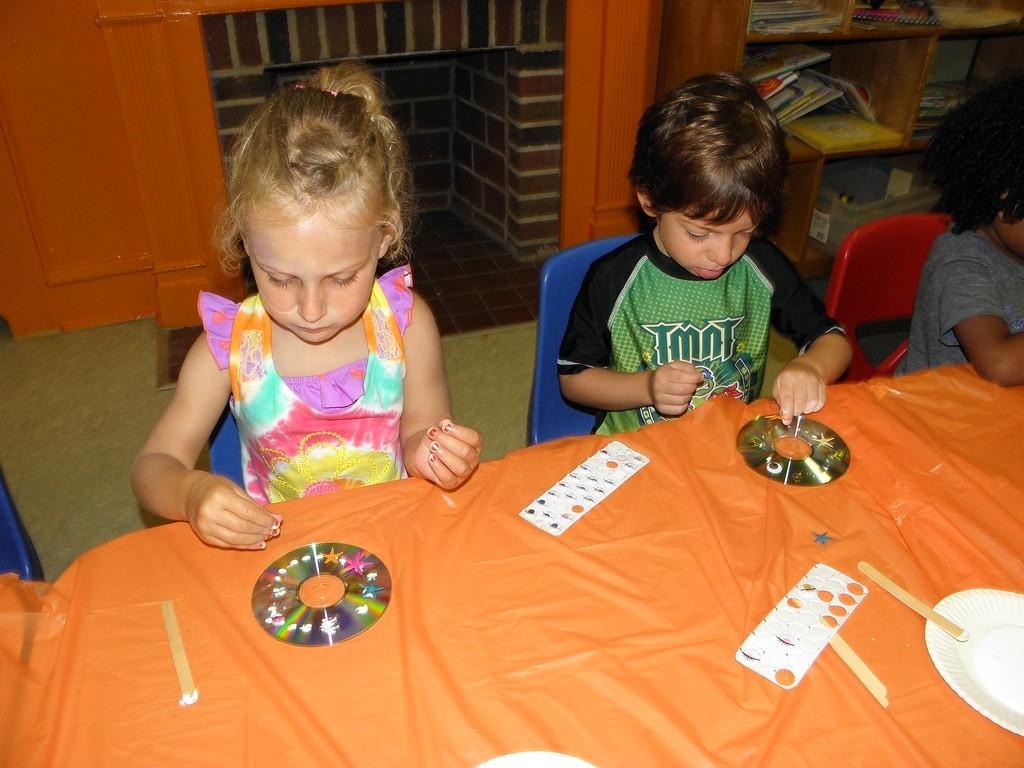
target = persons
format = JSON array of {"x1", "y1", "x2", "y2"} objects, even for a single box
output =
[
  {"x1": 558, "y1": 73, "x2": 852, "y2": 428},
  {"x1": 131, "y1": 64, "x2": 478, "y2": 552},
  {"x1": 904, "y1": 69, "x2": 1024, "y2": 385}
]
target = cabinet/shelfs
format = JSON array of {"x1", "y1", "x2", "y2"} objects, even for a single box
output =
[{"x1": 662, "y1": 0, "x2": 1024, "y2": 284}]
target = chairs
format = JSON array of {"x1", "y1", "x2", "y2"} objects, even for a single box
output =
[
  {"x1": 526, "y1": 234, "x2": 686, "y2": 446},
  {"x1": 824, "y1": 212, "x2": 957, "y2": 382}
]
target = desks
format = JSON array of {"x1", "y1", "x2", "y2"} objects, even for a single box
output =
[{"x1": 0, "y1": 338, "x2": 1024, "y2": 768}]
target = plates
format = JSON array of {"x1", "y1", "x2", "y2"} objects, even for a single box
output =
[
  {"x1": 924, "y1": 589, "x2": 1024, "y2": 736},
  {"x1": 474, "y1": 751, "x2": 598, "y2": 768}
]
